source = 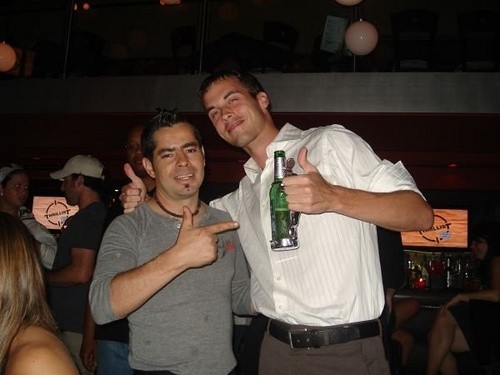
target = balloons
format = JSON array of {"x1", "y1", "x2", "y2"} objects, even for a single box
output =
[
  {"x1": 334, "y1": 0, "x2": 361, "y2": 7},
  {"x1": 347, "y1": 19, "x2": 378, "y2": 56},
  {"x1": 0, "y1": 43, "x2": 16, "y2": 71}
]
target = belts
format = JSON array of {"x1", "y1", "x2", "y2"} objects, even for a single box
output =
[{"x1": 267, "y1": 319, "x2": 380, "y2": 350}]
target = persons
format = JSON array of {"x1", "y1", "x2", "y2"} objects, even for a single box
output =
[
  {"x1": 87, "y1": 108, "x2": 252, "y2": 375},
  {"x1": 0, "y1": 121, "x2": 215, "y2": 375},
  {"x1": 118, "y1": 67, "x2": 434, "y2": 375},
  {"x1": 374, "y1": 221, "x2": 500, "y2": 375},
  {"x1": 0, "y1": 212, "x2": 81, "y2": 375}
]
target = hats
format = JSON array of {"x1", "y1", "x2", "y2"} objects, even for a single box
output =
[
  {"x1": 0, "y1": 163, "x2": 23, "y2": 183},
  {"x1": 49, "y1": 155, "x2": 105, "y2": 181}
]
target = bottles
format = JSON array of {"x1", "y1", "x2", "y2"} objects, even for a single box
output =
[
  {"x1": 270, "y1": 150, "x2": 291, "y2": 242},
  {"x1": 407, "y1": 251, "x2": 477, "y2": 295}
]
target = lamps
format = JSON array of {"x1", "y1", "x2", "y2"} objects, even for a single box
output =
[
  {"x1": 0, "y1": 42, "x2": 17, "y2": 72},
  {"x1": 337, "y1": 0, "x2": 362, "y2": 6},
  {"x1": 345, "y1": 18, "x2": 378, "y2": 55}
]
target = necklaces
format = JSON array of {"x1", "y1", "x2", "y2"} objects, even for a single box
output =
[{"x1": 152, "y1": 192, "x2": 201, "y2": 218}]
